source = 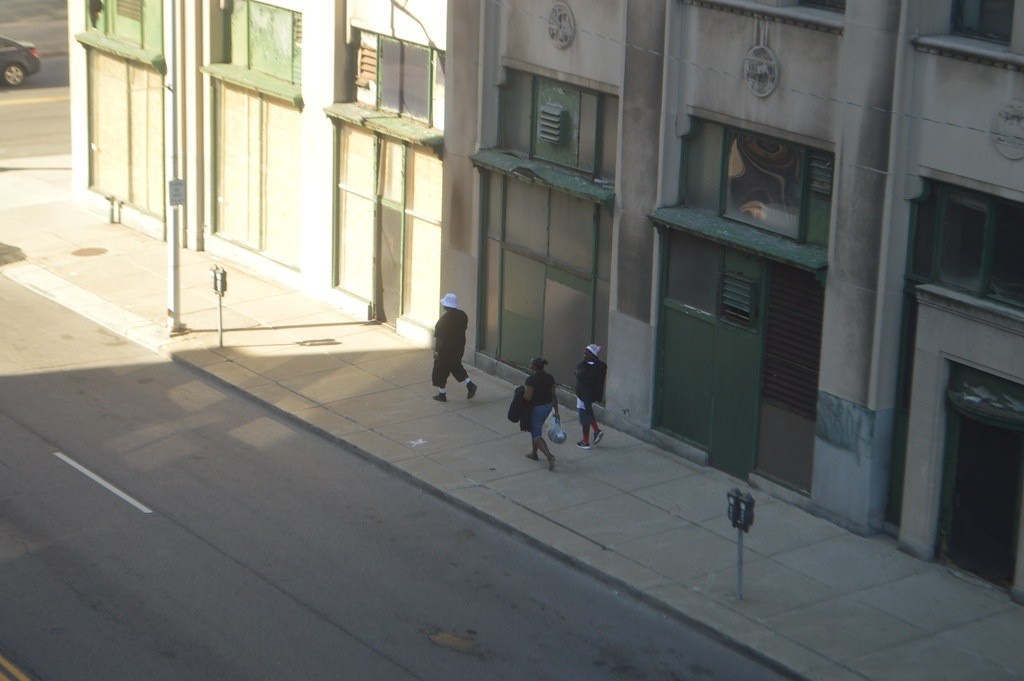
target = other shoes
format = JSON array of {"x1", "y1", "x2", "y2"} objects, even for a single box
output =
[
  {"x1": 525, "y1": 452, "x2": 538, "y2": 460},
  {"x1": 547, "y1": 453, "x2": 555, "y2": 470},
  {"x1": 466, "y1": 381, "x2": 477, "y2": 398},
  {"x1": 433, "y1": 392, "x2": 446, "y2": 400}
]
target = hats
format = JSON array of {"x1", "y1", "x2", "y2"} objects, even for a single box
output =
[
  {"x1": 440, "y1": 292, "x2": 458, "y2": 308},
  {"x1": 586, "y1": 342, "x2": 602, "y2": 357},
  {"x1": 529, "y1": 357, "x2": 548, "y2": 369}
]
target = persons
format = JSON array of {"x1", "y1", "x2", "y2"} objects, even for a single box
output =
[
  {"x1": 431, "y1": 293, "x2": 477, "y2": 402},
  {"x1": 522, "y1": 356, "x2": 560, "y2": 471},
  {"x1": 574, "y1": 343, "x2": 608, "y2": 449}
]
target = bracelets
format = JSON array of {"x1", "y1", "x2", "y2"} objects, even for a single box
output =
[{"x1": 434, "y1": 352, "x2": 438, "y2": 356}]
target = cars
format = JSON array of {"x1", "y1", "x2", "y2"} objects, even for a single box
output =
[{"x1": 0, "y1": 32, "x2": 41, "y2": 88}]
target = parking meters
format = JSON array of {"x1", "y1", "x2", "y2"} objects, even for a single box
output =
[
  {"x1": 209, "y1": 264, "x2": 228, "y2": 348},
  {"x1": 725, "y1": 486, "x2": 756, "y2": 599}
]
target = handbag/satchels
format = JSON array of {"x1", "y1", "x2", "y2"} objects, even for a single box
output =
[
  {"x1": 432, "y1": 352, "x2": 446, "y2": 386},
  {"x1": 547, "y1": 417, "x2": 567, "y2": 444}
]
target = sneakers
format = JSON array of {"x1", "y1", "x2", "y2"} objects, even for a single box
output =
[
  {"x1": 592, "y1": 429, "x2": 603, "y2": 443},
  {"x1": 576, "y1": 440, "x2": 591, "y2": 448}
]
